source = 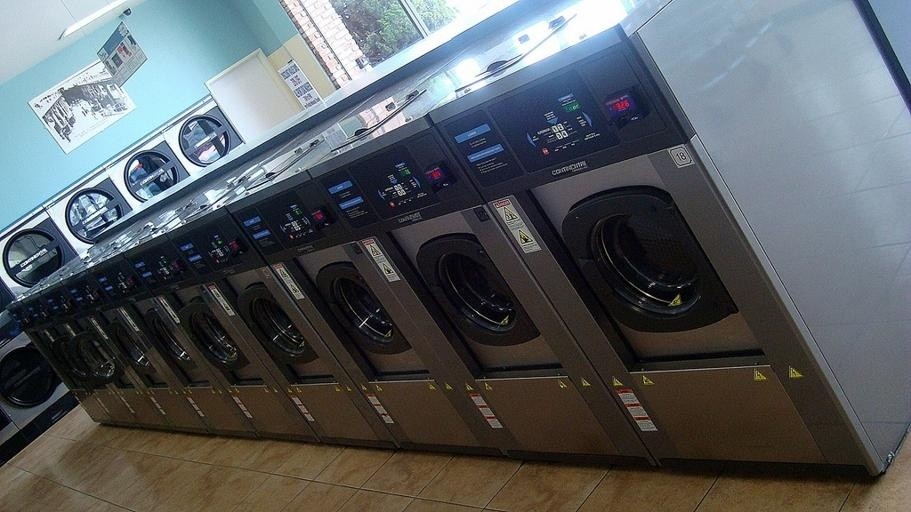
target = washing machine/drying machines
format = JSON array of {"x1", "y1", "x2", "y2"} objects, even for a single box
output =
[{"x1": 1, "y1": 2, "x2": 911, "y2": 483}]
